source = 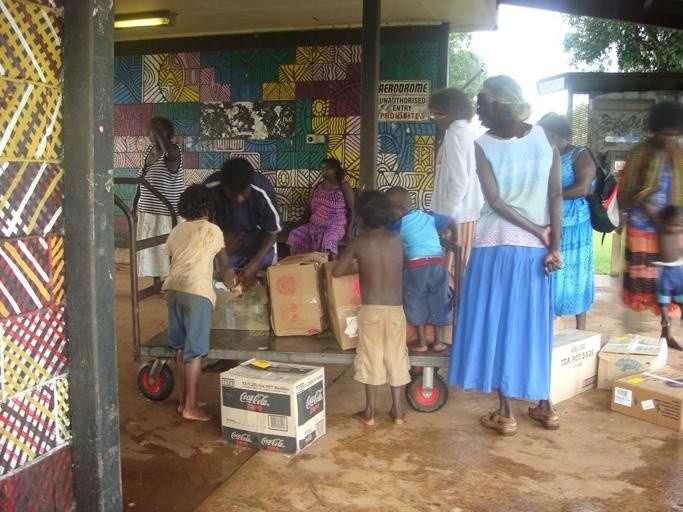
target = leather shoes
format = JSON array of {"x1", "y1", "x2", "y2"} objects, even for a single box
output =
[{"x1": 206, "y1": 359, "x2": 241, "y2": 372}]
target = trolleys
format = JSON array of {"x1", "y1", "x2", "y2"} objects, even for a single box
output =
[{"x1": 111, "y1": 172, "x2": 459, "y2": 417}]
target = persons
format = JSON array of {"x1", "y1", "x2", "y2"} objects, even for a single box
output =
[
  {"x1": 196, "y1": 158, "x2": 282, "y2": 372},
  {"x1": 613, "y1": 100, "x2": 683, "y2": 352},
  {"x1": 385, "y1": 184, "x2": 457, "y2": 352},
  {"x1": 443, "y1": 75, "x2": 564, "y2": 435},
  {"x1": 159, "y1": 183, "x2": 239, "y2": 422},
  {"x1": 630, "y1": 195, "x2": 683, "y2": 326},
  {"x1": 425, "y1": 85, "x2": 484, "y2": 305},
  {"x1": 127, "y1": 117, "x2": 188, "y2": 295},
  {"x1": 330, "y1": 188, "x2": 412, "y2": 425},
  {"x1": 536, "y1": 110, "x2": 597, "y2": 331},
  {"x1": 283, "y1": 153, "x2": 358, "y2": 261}
]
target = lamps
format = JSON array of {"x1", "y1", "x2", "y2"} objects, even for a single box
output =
[{"x1": 110, "y1": 8, "x2": 179, "y2": 33}]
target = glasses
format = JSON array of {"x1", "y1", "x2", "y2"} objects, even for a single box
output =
[{"x1": 429, "y1": 112, "x2": 456, "y2": 120}]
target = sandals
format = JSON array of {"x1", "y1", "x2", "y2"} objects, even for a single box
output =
[
  {"x1": 480, "y1": 408, "x2": 517, "y2": 436},
  {"x1": 528, "y1": 407, "x2": 561, "y2": 430}
]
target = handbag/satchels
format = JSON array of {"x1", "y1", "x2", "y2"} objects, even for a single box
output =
[{"x1": 572, "y1": 146, "x2": 620, "y2": 232}]
